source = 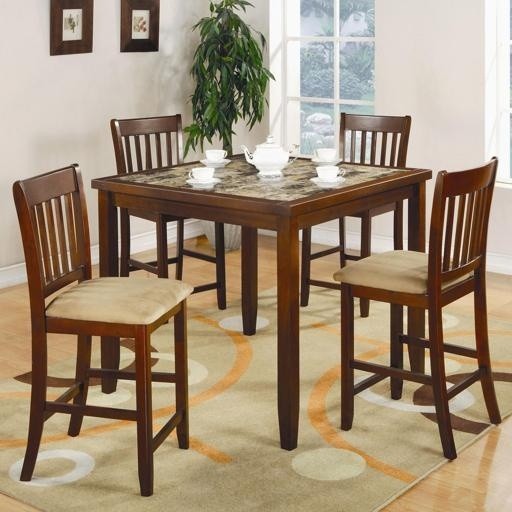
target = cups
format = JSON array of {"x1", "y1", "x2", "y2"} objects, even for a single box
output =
[
  {"x1": 316, "y1": 167, "x2": 345, "y2": 181},
  {"x1": 317, "y1": 148, "x2": 337, "y2": 161},
  {"x1": 205, "y1": 150, "x2": 227, "y2": 161},
  {"x1": 188, "y1": 167, "x2": 216, "y2": 181}
]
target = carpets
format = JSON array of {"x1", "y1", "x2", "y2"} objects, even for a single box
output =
[{"x1": 0, "y1": 283, "x2": 511, "y2": 511}]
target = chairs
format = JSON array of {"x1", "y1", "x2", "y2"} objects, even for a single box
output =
[
  {"x1": 299, "y1": 110, "x2": 413, "y2": 319},
  {"x1": 330, "y1": 153, "x2": 502, "y2": 461},
  {"x1": 12, "y1": 161, "x2": 197, "y2": 500},
  {"x1": 109, "y1": 112, "x2": 228, "y2": 326}
]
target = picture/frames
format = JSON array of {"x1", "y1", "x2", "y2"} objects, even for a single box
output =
[
  {"x1": 119, "y1": 1, "x2": 162, "y2": 55},
  {"x1": 49, "y1": 0, "x2": 96, "y2": 58}
]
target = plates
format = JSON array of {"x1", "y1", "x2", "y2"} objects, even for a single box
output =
[
  {"x1": 186, "y1": 178, "x2": 222, "y2": 190},
  {"x1": 310, "y1": 175, "x2": 343, "y2": 189},
  {"x1": 199, "y1": 159, "x2": 231, "y2": 168},
  {"x1": 312, "y1": 158, "x2": 345, "y2": 166}
]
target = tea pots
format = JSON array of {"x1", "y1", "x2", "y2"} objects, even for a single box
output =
[{"x1": 242, "y1": 134, "x2": 298, "y2": 176}]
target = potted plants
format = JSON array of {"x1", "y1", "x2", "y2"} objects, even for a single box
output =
[{"x1": 181, "y1": 0, "x2": 276, "y2": 254}]
